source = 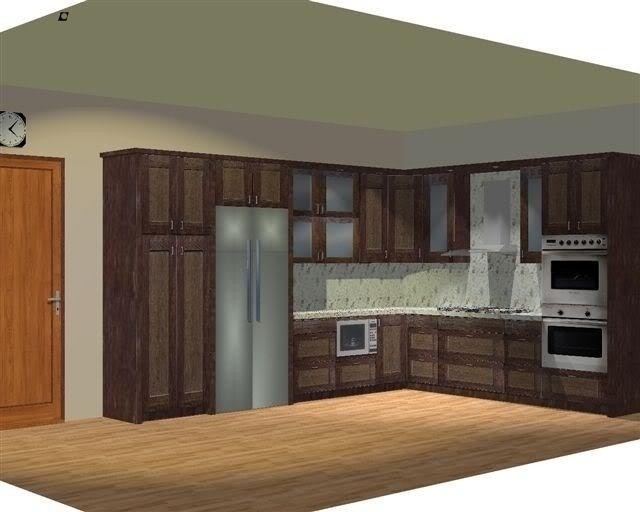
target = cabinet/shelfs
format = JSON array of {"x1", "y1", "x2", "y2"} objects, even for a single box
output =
[
  {"x1": 217, "y1": 157, "x2": 288, "y2": 208},
  {"x1": 362, "y1": 165, "x2": 471, "y2": 260},
  {"x1": 542, "y1": 152, "x2": 637, "y2": 231},
  {"x1": 98, "y1": 149, "x2": 217, "y2": 424},
  {"x1": 294, "y1": 318, "x2": 335, "y2": 402},
  {"x1": 520, "y1": 164, "x2": 543, "y2": 263},
  {"x1": 289, "y1": 162, "x2": 360, "y2": 264},
  {"x1": 409, "y1": 315, "x2": 542, "y2": 408},
  {"x1": 379, "y1": 316, "x2": 409, "y2": 390}
]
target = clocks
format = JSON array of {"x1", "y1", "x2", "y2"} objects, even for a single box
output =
[{"x1": 0, "y1": 111, "x2": 28, "y2": 148}]
